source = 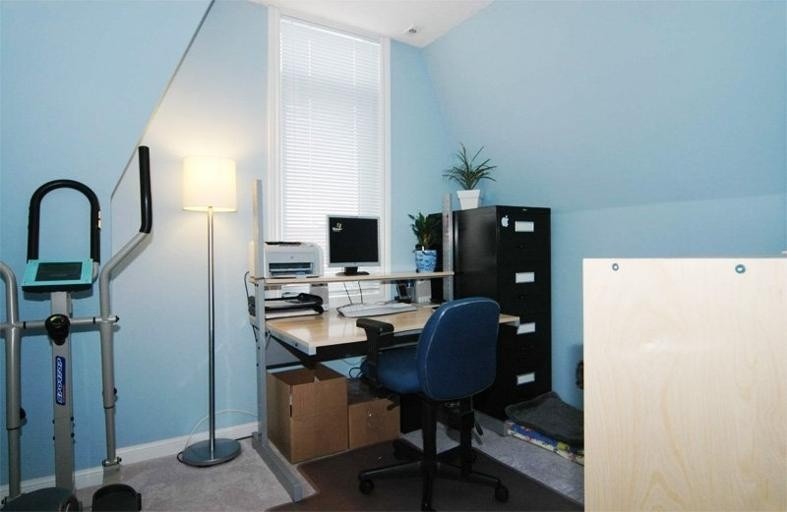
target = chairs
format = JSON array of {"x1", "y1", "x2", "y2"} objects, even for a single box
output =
[{"x1": 358, "y1": 291, "x2": 510, "y2": 511}]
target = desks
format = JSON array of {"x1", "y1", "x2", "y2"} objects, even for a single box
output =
[{"x1": 248, "y1": 308, "x2": 521, "y2": 505}]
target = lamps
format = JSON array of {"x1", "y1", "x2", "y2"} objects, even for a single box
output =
[{"x1": 176, "y1": 154, "x2": 243, "y2": 470}]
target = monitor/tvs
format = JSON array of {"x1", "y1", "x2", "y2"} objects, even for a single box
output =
[{"x1": 326, "y1": 213, "x2": 382, "y2": 277}]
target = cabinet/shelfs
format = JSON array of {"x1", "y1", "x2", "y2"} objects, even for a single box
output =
[{"x1": 453, "y1": 205, "x2": 551, "y2": 420}]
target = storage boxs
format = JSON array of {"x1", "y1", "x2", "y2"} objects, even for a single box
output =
[{"x1": 267, "y1": 365, "x2": 402, "y2": 464}]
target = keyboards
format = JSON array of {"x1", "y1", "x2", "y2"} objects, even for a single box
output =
[{"x1": 336, "y1": 300, "x2": 417, "y2": 318}]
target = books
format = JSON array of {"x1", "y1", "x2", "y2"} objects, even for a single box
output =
[{"x1": 416, "y1": 279, "x2": 432, "y2": 304}]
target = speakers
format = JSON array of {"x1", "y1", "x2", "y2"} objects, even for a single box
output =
[
  {"x1": 310, "y1": 284, "x2": 329, "y2": 311},
  {"x1": 411, "y1": 280, "x2": 432, "y2": 303}
]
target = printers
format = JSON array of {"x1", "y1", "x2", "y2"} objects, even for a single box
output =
[{"x1": 247, "y1": 239, "x2": 324, "y2": 279}]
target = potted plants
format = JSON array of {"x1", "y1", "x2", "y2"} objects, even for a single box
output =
[
  {"x1": 407, "y1": 210, "x2": 441, "y2": 274},
  {"x1": 439, "y1": 140, "x2": 496, "y2": 210}
]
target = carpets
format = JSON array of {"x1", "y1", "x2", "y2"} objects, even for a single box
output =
[{"x1": 263, "y1": 438, "x2": 584, "y2": 512}]
